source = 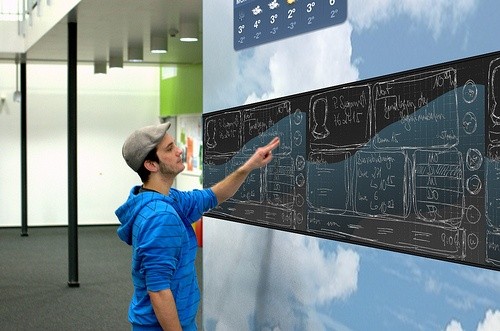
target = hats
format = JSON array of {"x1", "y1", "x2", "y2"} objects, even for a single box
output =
[{"x1": 122, "y1": 122, "x2": 171, "y2": 172}]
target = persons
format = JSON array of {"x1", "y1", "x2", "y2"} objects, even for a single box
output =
[{"x1": 115, "y1": 123, "x2": 280, "y2": 331}]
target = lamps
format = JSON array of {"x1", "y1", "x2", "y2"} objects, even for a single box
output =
[
  {"x1": 94, "y1": 60, "x2": 106, "y2": 74},
  {"x1": 13, "y1": 59, "x2": 22, "y2": 102},
  {"x1": 150, "y1": 34, "x2": 167, "y2": 53},
  {"x1": 110, "y1": 47, "x2": 123, "y2": 68},
  {"x1": 179, "y1": 22, "x2": 198, "y2": 42},
  {"x1": 127, "y1": 39, "x2": 143, "y2": 61}
]
